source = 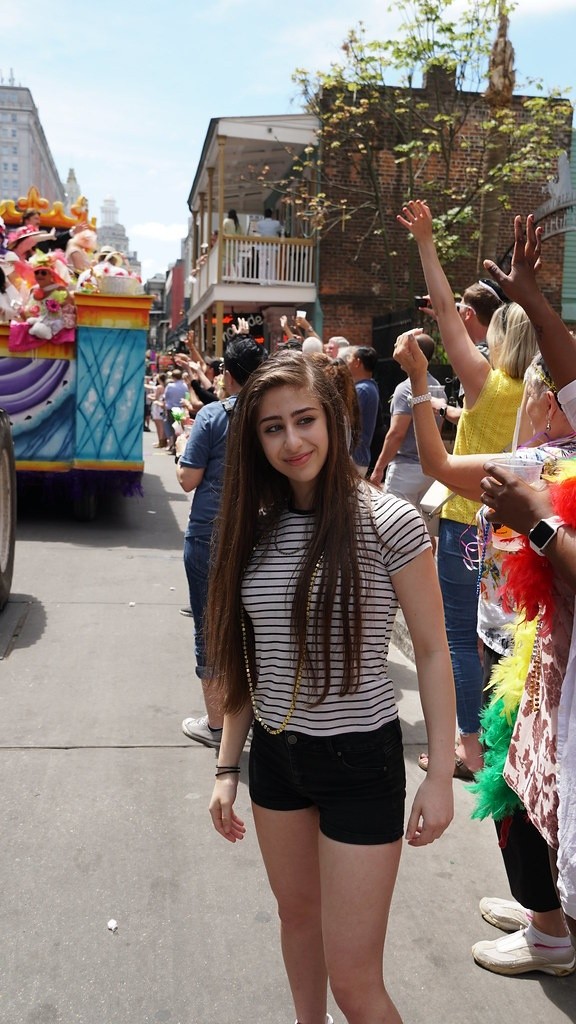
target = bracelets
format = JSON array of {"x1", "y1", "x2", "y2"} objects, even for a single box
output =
[{"x1": 214, "y1": 764, "x2": 243, "y2": 778}]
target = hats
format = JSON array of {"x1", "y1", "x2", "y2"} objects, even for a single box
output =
[
  {"x1": 205, "y1": 355, "x2": 223, "y2": 370},
  {"x1": 94, "y1": 245, "x2": 123, "y2": 267},
  {"x1": 28, "y1": 247, "x2": 71, "y2": 287},
  {"x1": 7, "y1": 223, "x2": 49, "y2": 244}
]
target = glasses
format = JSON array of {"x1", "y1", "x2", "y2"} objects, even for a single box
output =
[
  {"x1": 35, "y1": 270, "x2": 50, "y2": 277},
  {"x1": 455, "y1": 303, "x2": 477, "y2": 316}
]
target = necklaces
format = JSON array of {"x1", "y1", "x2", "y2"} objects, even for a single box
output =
[
  {"x1": 232, "y1": 470, "x2": 354, "y2": 737},
  {"x1": 273, "y1": 505, "x2": 316, "y2": 556}
]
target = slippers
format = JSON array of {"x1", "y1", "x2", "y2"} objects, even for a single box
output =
[{"x1": 417, "y1": 750, "x2": 477, "y2": 780}]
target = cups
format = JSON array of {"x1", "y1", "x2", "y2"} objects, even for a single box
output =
[
  {"x1": 296, "y1": 310, "x2": 307, "y2": 320},
  {"x1": 489, "y1": 458, "x2": 543, "y2": 552},
  {"x1": 428, "y1": 386, "x2": 445, "y2": 416},
  {"x1": 183, "y1": 425, "x2": 193, "y2": 440}
]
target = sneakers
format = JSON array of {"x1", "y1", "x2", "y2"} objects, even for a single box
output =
[
  {"x1": 182, "y1": 713, "x2": 223, "y2": 749},
  {"x1": 478, "y1": 896, "x2": 534, "y2": 931},
  {"x1": 471, "y1": 927, "x2": 576, "y2": 977}
]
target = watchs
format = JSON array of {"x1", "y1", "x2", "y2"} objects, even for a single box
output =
[
  {"x1": 528, "y1": 515, "x2": 566, "y2": 557},
  {"x1": 406, "y1": 391, "x2": 430, "y2": 408},
  {"x1": 439, "y1": 403, "x2": 448, "y2": 418}
]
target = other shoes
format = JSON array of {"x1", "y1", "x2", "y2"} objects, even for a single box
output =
[
  {"x1": 152, "y1": 438, "x2": 167, "y2": 448},
  {"x1": 144, "y1": 426, "x2": 151, "y2": 432}
]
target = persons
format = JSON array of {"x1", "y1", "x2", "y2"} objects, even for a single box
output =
[
  {"x1": 367, "y1": 326, "x2": 450, "y2": 558},
  {"x1": 478, "y1": 213, "x2": 576, "y2": 946},
  {"x1": 391, "y1": 329, "x2": 576, "y2": 980},
  {"x1": 144, "y1": 203, "x2": 381, "y2": 479},
  {"x1": 394, "y1": 198, "x2": 539, "y2": 780},
  {"x1": 0, "y1": 208, "x2": 121, "y2": 323},
  {"x1": 414, "y1": 279, "x2": 500, "y2": 428},
  {"x1": 140, "y1": 351, "x2": 463, "y2": 1023}
]
